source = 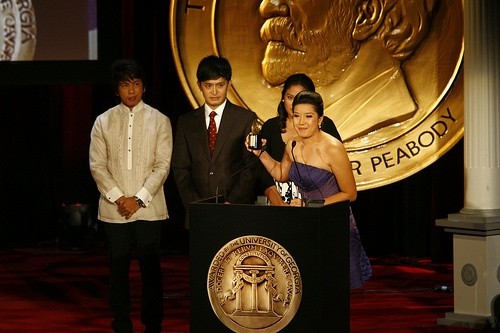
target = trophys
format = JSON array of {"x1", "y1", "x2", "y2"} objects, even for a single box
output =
[{"x1": 249, "y1": 119, "x2": 263, "y2": 150}]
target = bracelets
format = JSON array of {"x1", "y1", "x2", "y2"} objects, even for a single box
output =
[{"x1": 258, "y1": 149, "x2": 265, "y2": 157}]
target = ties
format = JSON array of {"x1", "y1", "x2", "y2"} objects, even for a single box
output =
[{"x1": 207, "y1": 111, "x2": 218, "y2": 157}]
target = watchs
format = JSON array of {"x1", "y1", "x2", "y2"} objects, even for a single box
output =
[{"x1": 134, "y1": 196, "x2": 145, "y2": 208}]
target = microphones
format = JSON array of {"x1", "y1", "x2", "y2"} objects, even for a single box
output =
[
  {"x1": 216, "y1": 145, "x2": 268, "y2": 204},
  {"x1": 292, "y1": 141, "x2": 304, "y2": 207}
]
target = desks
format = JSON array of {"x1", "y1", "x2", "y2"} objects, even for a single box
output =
[{"x1": 189, "y1": 194, "x2": 350, "y2": 333}]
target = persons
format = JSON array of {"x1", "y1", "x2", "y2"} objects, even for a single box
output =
[
  {"x1": 259, "y1": 0, "x2": 436, "y2": 144},
  {"x1": 244, "y1": 91, "x2": 372, "y2": 292},
  {"x1": 171, "y1": 56, "x2": 263, "y2": 229},
  {"x1": 253, "y1": 74, "x2": 342, "y2": 206},
  {"x1": 89, "y1": 62, "x2": 173, "y2": 333}
]
